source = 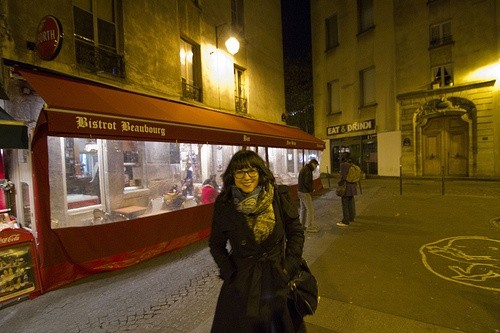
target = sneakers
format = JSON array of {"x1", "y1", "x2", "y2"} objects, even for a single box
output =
[{"x1": 336, "y1": 223, "x2": 349, "y2": 226}]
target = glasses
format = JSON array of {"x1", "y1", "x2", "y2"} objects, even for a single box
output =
[{"x1": 233, "y1": 168, "x2": 259, "y2": 179}]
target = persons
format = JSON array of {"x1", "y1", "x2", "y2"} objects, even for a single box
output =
[
  {"x1": 336, "y1": 151, "x2": 362, "y2": 227},
  {"x1": 201, "y1": 173, "x2": 228, "y2": 204},
  {"x1": 185, "y1": 161, "x2": 194, "y2": 196},
  {"x1": 297, "y1": 158, "x2": 320, "y2": 233}
]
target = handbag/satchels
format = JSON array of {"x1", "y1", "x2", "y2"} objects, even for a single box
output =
[
  {"x1": 336, "y1": 185, "x2": 346, "y2": 196},
  {"x1": 284, "y1": 248, "x2": 318, "y2": 317}
]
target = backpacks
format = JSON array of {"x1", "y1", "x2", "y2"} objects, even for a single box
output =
[{"x1": 345, "y1": 163, "x2": 361, "y2": 182}]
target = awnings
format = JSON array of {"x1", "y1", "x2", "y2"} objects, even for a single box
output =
[
  {"x1": 1, "y1": 65, "x2": 326, "y2": 201},
  {"x1": 0, "y1": 106, "x2": 30, "y2": 150},
  {"x1": 208, "y1": 150, "x2": 305, "y2": 333}
]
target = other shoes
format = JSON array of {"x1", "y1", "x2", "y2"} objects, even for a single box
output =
[{"x1": 306, "y1": 229, "x2": 318, "y2": 232}]
